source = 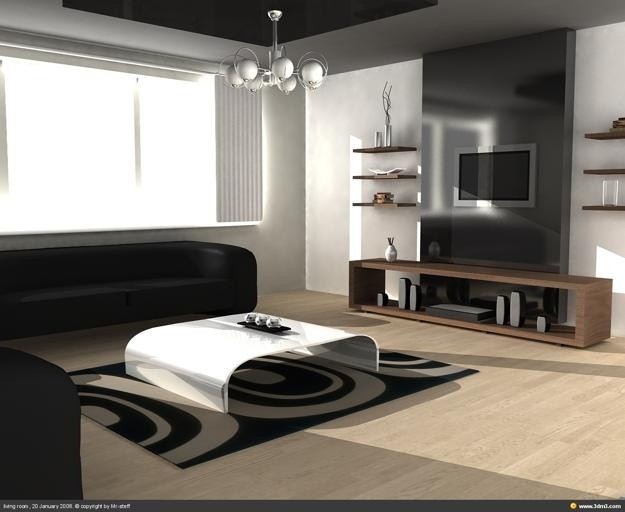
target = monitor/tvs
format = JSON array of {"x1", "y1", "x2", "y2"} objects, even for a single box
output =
[{"x1": 453, "y1": 143, "x2": 538, "y2": 209}]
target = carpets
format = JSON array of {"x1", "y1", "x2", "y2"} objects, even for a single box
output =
[{"x1": 65, "y1": 346, "x2": 480, "y2": 470}]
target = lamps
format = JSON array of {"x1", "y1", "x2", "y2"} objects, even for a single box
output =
[{"x1": 218, "y1": 9, "x2": 329, "y2": 97}]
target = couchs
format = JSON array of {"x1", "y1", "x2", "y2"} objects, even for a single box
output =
[
  {"x1": 0, "y1": 345, "x2": 86, "y2": 500},
  {"x1": 0, "y1": 241, "x2": 258, "y2": 341}
]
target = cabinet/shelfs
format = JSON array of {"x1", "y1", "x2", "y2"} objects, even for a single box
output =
[
  {"x1": 582, "y1": 131, "x2": 625, "y2": 211},
  {"x1": 348, "y1": 257, "x2": 613, "y2": 349},
  {"x1": 352, "y1": 146, "x2": 417, "y2": 207}
]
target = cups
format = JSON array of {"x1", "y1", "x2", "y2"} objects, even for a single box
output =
[{"x1": 602, "y1": 180, "x2": 619, "y2": 207}]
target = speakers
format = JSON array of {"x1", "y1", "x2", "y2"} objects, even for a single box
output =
[
  {"x1": 510, "y1": 291, "x2": 526, "y2": 328},
  {"x1": 537, "y1": 316, "x2": 550, "y2": 332},
  {"x1": 496, "y1": 295, "x2": 509, "y2": 324},
  {"x1": 409, "y1": 284, "x2": 422, "y2": 310},
  {"x1": 377, "y1": 293, "x2": 388, "y2": 305},
  {"x1": 398, "y1": 278, "x2": 412, "y2": 310}
]
